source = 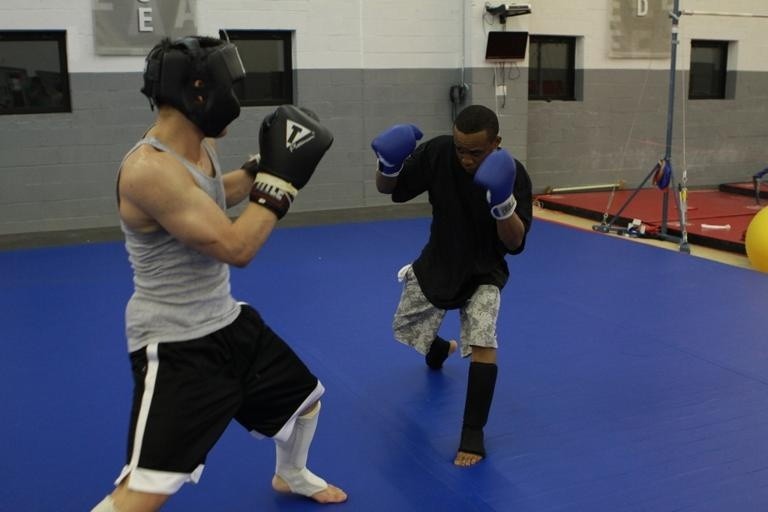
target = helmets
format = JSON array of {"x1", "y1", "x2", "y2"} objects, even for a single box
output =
[{"x1": 144, "y1": 30, "x2": 246, "y2": 136}]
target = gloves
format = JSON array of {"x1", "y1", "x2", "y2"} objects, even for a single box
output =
[
  {"x1": 473, "y1": 150, "x2": 516, "y2": 220},
  {"x1": 371, "y1": 123, "x2": 423, "y2": 177},
  {"x1": 242, "y1": 105, "x2": 332, "y2": 219}
]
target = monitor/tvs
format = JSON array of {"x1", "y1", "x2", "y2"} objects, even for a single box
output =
[{"x1": 484, "y1": 30, "x2": 529, "y2": 63}]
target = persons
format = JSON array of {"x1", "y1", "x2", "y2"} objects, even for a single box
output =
[
  {"x1": 88, "y1": 35, "x2": 351, "y2": 511},
  {"x1": 370, "y1": 103, "x2": 535, "y2": 467}
]
